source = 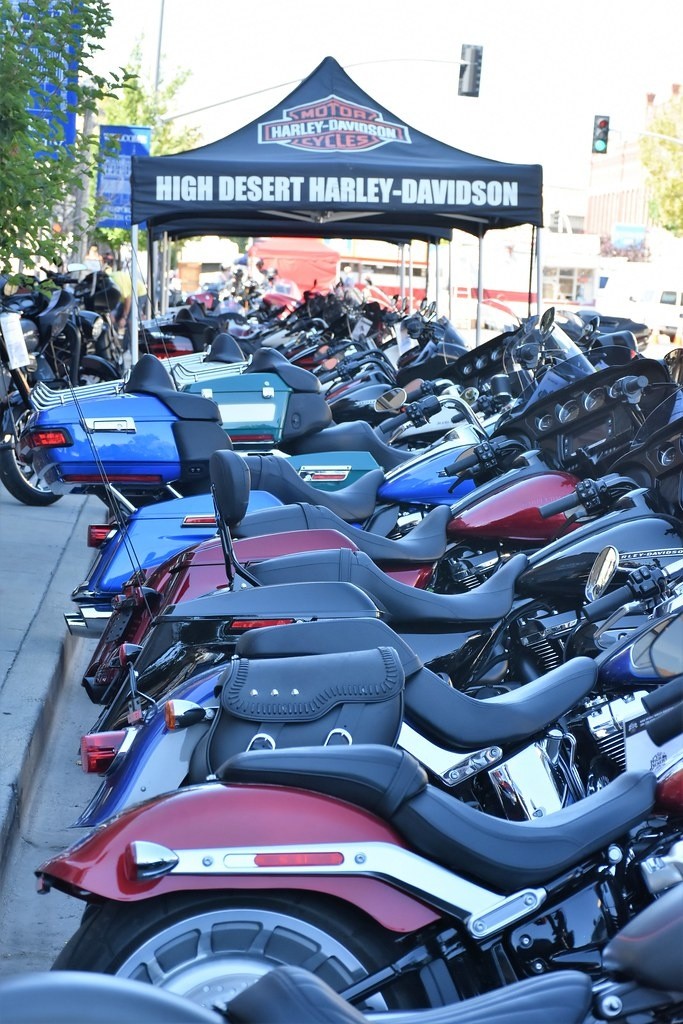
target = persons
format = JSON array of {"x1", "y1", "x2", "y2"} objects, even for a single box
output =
[{"x1": 80, "y1": 246, "x2": 148, "y2": 353}]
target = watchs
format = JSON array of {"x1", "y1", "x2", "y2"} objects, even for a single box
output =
[{"x1": 122, "y1": 314, "x2": 128, "y2": 320}]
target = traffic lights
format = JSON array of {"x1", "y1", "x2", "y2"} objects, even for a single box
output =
[{"x1": 592, "y1": 115, "x2": 609, "y2": 153}]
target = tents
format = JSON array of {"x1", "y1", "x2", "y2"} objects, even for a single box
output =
[{"x1": 130, "y1": 56, "x2": 543, "y2": 368}]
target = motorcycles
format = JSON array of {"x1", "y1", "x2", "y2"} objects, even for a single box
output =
[
  {"x1": 0, "y1": 258, "x2": 683, "y2": 595},
  {"x1": 0, "y1": 876, "x2": 683, "y2": 1024},
  {"x1": 66, "y1": 544, "x2": 683, "y2": 822},
  {"x1": 32, "y1": 685, "x2": 683, "y2": 1020}
]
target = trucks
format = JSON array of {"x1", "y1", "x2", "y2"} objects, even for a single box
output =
[{"x1": 596, "y1": 261, "x2": 683, "y2": 334}]
